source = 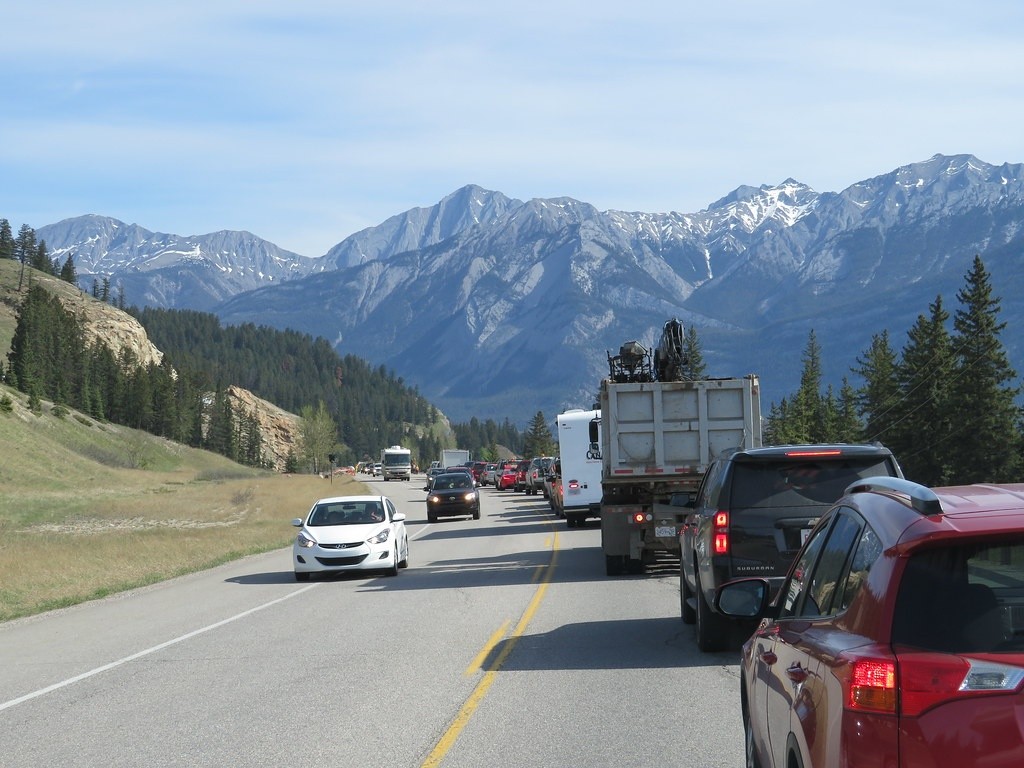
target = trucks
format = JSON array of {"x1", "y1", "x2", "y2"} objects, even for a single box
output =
[
  {"x1": 556, "y1": 408, "x2": 605, "y2": 526},
  {"x1": 586, "y1": 375, "x2": 762, "y2": 579}
]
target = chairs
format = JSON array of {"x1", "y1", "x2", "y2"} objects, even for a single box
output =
[
  {"x1": 327, "y1": 511, "x2": 345, "y2": 522},
  {"x1": 968, "y1": 583, "x2": 997, "y2": 619}
]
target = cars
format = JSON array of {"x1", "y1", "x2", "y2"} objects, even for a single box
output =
[
  {"x1": 424, "y1": 449, "x2": 564, "y2": 519},
  {"x1": 356, "y1": 460, "x2": 382, "y2": 477},
  {"x1": 292, "y1": 496, "x2": 409, "y2": 583},
  {"x1": 425, "y1": 471, "x2": 482, "y2": 523}
]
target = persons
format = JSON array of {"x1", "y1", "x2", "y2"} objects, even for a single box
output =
[
  {"x1": 357, "y1": 502, "x2": 383, "y2": 522},
  {"x1": 411, "y1": 458, "x2": 419, "y2": 474},
  {"x1": 457, "y1": 480, "x2": 468, "y2": 488}
]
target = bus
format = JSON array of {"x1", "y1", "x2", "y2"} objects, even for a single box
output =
[{"x1": 380, "y1": 446, "x2": 411, "y2": 482}]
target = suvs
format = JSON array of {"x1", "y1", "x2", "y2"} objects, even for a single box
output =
[
  {"x1": 668, "y1": 441, "x2": 907, "y2": 652},
  {"x1": 709, "y1": 476, "x2": 1024, "y2": 768}
]
